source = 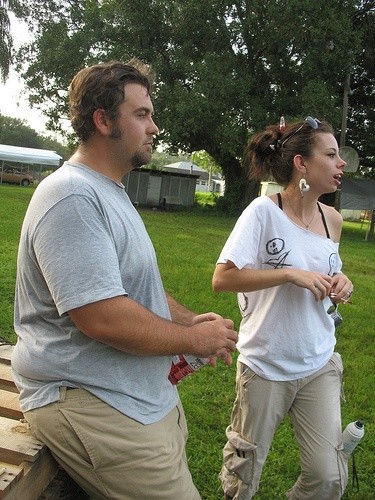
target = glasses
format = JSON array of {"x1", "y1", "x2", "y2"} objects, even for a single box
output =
[{"x1": 281, "y1": 116, "x2": 322, "y2": 158}]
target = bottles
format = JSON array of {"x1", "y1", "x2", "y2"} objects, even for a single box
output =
[
  {"x1": 167, "y1": 340, "x2": 223, "y2": 388},
  {"x1": 343, "y1": 418, "x2": 365, "y2": 457}
]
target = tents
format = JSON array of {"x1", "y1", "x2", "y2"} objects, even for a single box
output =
[{"x1": 0, "y1": 143, "x2": 62, "y2": 188}]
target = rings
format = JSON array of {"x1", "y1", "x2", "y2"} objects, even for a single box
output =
[{"x1": 347, "y1": 289, "x2": 352, "y2": 296}]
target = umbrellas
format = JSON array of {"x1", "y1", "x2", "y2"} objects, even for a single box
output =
[{"x1": 163, "y1": 161, "x2": 207, "y2": 176}]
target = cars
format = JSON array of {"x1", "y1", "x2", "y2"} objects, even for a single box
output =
[{"x1": 0, "y1": 166, "x2": 34, "y2": 187}]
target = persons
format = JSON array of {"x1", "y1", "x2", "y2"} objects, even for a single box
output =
[
  {"x1": 11, "y1": 60, "x2": 242, "y2": 500},
  {"x1": 211, "y1": 115, "x2": 356, "y2": 500}
]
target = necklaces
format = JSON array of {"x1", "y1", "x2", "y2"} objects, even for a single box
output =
[{"x1": 285, "y1": 189, "x2": 318, "y2": 230}]
